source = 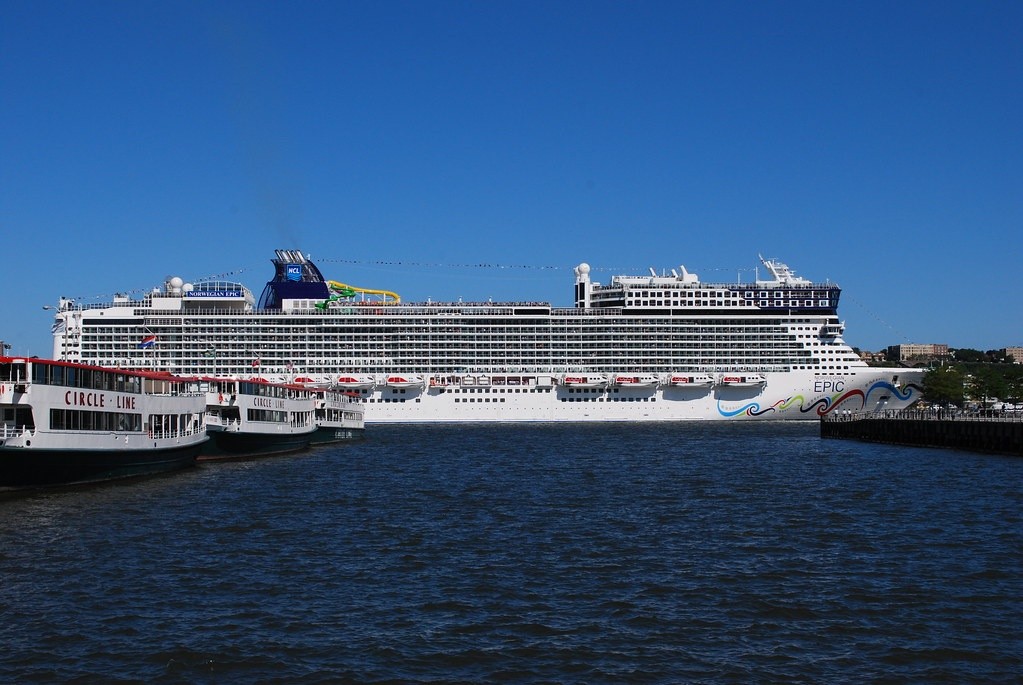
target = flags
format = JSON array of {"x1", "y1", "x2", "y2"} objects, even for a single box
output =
[
  {"x1": 203, "y1": 348, "x2": 215, "y2": 357},
  {"x1": 287, "y1": 363, "x2": 293, "y2": 369},
  {"x1": 252, "y1": 358, "x2": 260, "y2": 367},
  {"x1": 138, "y1": 335, "x2": 155, "y2": 348}
]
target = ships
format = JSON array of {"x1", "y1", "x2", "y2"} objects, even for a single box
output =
[{"x1": 52, "y1": 249, "x2": 931, "y2": 423}]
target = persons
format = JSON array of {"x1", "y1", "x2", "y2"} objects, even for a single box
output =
[
  {"x1": 854, "y1": 407, "x2": 858, "y2": 419},
  {"x1": 834, "y1": 408, "x2": 838, "y2": 421},
  {"x1": 843, "y1": 409, "x2": 847, "y2": 421},
  {"x1": 1001, "y1": 404, "x2": 1006, "y2": 417},
  {"x1": 848, "y1": 409, "x2": 851, "y2": 421}
]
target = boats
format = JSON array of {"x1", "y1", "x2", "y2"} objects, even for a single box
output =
[
  {"x1": 616, "y1": 375, "x2": 659, "y2": 388},
  {"x1": 671, "y1": 375, "x2": 714, "y2": 386},
  {"x1": 250, "y1": 376, "x2": 287, "y2": 387},
  {"x1": 387, "y1": 377, "x2": 424, "y2": 389},
  {"x1": 723, "y1": 374, "x2": 765, "y2": 387},
  {"x1": 293, "y1": 377, "x2": 331, "y2": 388},
  {"x1": 564, "y1": 374, "x2": 609, "y2": 387},
  {"x1": 339, "y1": 376, "x2": 374, "y2": 389},
  {"x1": 0, "y1": 352, "x2": 369, "y2": 493},
  {"x1": 1015, "y1": 402, "x2": 1023, "y2": 418},
  {"x1": 974, "y1": 396, "x2": 1015, "y2": 416}
]
